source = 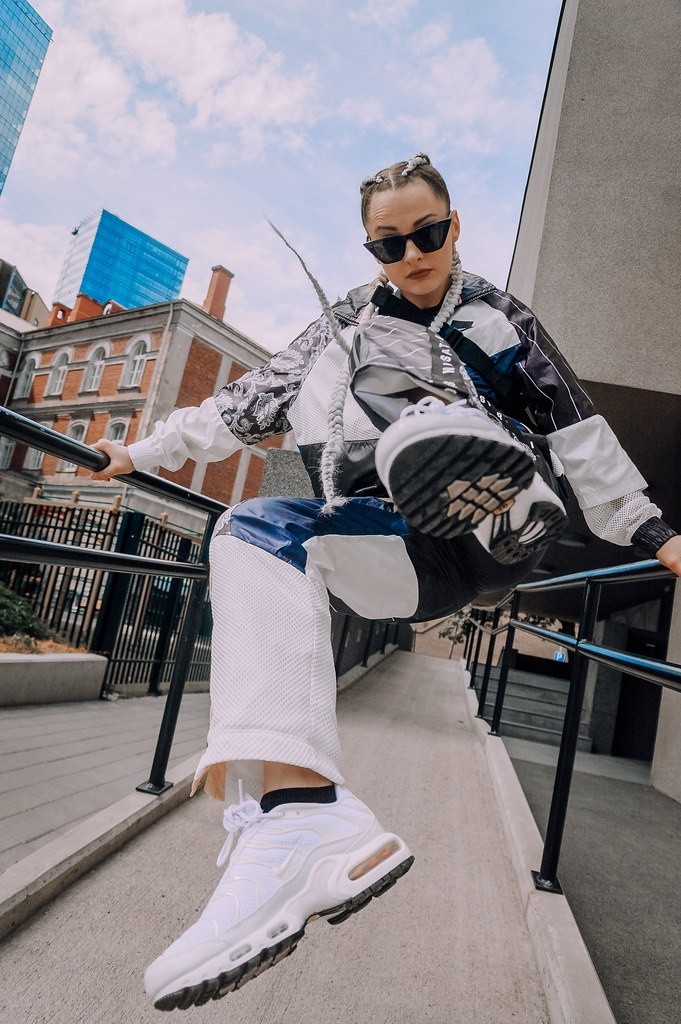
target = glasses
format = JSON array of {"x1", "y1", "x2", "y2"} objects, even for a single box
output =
[{"x1": 363, "y1": 210, "x2": 452, "y2": 264}]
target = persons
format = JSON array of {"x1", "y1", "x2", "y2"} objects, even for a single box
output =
[{"x1": 88, "y1": 154, "x2": 681, "y2": 1011}]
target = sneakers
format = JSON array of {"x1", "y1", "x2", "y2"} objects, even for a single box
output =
[
  {"x1": 375, "y1": 396, "x2": 569, "y2": 565},
  {"x1": 144, "y1": 779, "x2": 414, "y2": 1011}
]
